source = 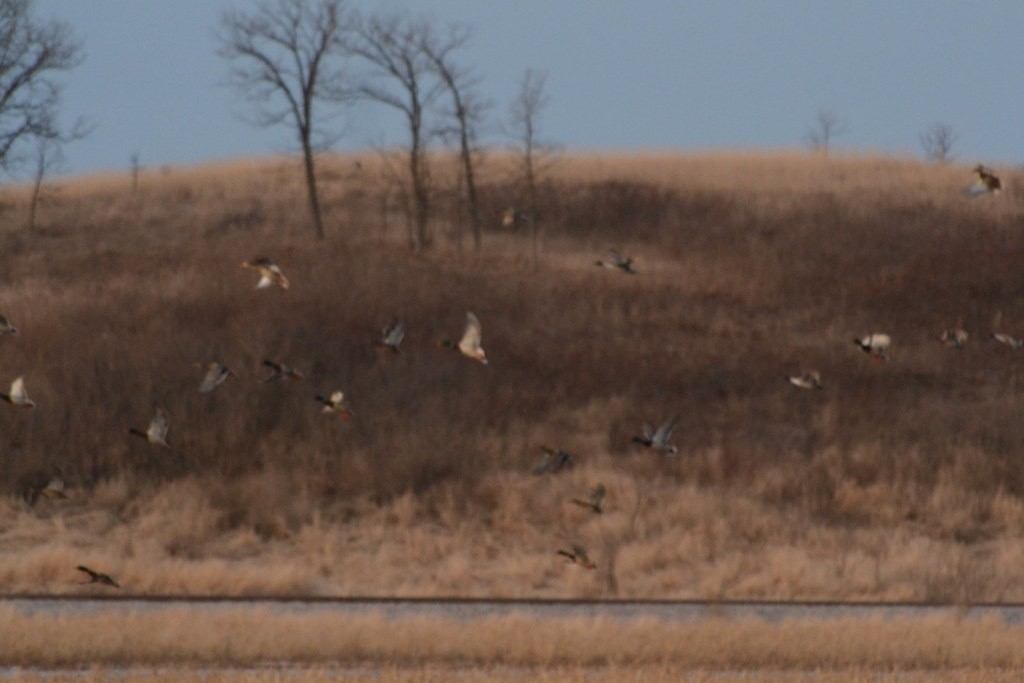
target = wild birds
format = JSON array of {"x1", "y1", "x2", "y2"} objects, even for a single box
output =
[
  {"x1": 592, "y1": 246, "x2": 641, "y2": 277},
  {"x1": 960, "y1": 163, "x2": 1003, "y2": 198},
  {"x1": 0, "y1": 374, "x2": 36, "y2": 409},
  {"x1": 248, "y1": 254, "x2": 292, "y2": 291},
  {"x1": 529, "y1": 413, "x2": 681, "y2": 571},
  {"x1": 781, "y1": 325, "x2": 1023, "y2": 391},
  {"x1": 119, "y1": 310, "x2": 490, "y2": 452},
  {"x1": 73, "y1": 564, "x2": 120, "y2": 588}
]
